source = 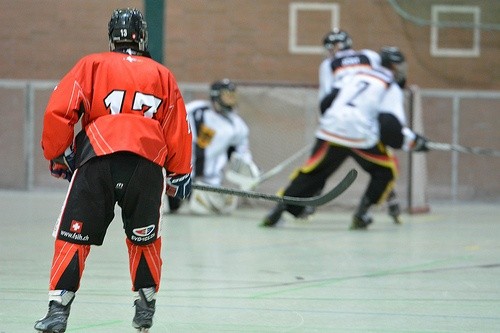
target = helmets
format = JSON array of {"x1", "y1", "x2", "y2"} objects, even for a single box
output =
[
  {"x1": 323, "y1": 29, "x2": 353, "y2": 54},
  {"x1": 108, "y1": 8, "x2": 149, "y2": 54},
  {"x1": 211, "y1": 79, "x2": 237, "y2": 116}
]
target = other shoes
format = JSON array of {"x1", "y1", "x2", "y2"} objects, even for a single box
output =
[
  {"x1": 163, "y1": 195, "x2": 181, "y2": 212},
  {"x1": 350, "y1": 213, "x2": 374, "y2": 231},
  {"x1": 263, "y1": 207, "x2": 281, "y2": 226}
]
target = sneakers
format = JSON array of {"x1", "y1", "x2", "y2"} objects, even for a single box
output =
[
  {"x1": 132, "y1": 287, "x2": 156, "y2": 333},
  {"x1": 33, "y1": 290, "x2": 76, "y2": 333}
]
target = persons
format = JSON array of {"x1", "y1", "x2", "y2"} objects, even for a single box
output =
[
  {"x1": 264, "y1": 46, "x2": 430, "y2": 230},
  {"x1": 186, "y1": 78, "x2": 251, "y2": 183},
  {"x1": 34, "y1": 9, "x2": 192, "y2": 333},
  {"x1": 301, "y1": 31, "x2": 400, "y2": 223}
]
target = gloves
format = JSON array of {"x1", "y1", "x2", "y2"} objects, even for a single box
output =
[
  {"x1": 166, "y1": 169, "x2": 191, "y2": 200},
  {"x1": 411, "y1": 134, "x2": 429, "y2": 153},
  {"x1": 49, "y1": 144, "x2": 73, "y2": 182}
]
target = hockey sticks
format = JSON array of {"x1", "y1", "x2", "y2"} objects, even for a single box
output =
[
  {"x1": 225, "y1": 137, "x2": 316, "y2": 187},
  {"x1": 427, "y1": 142, "x2": 500, "y2": 157},
  {"x1": 191, "y1": 168, "x2": 358, "y2": 206}
]
